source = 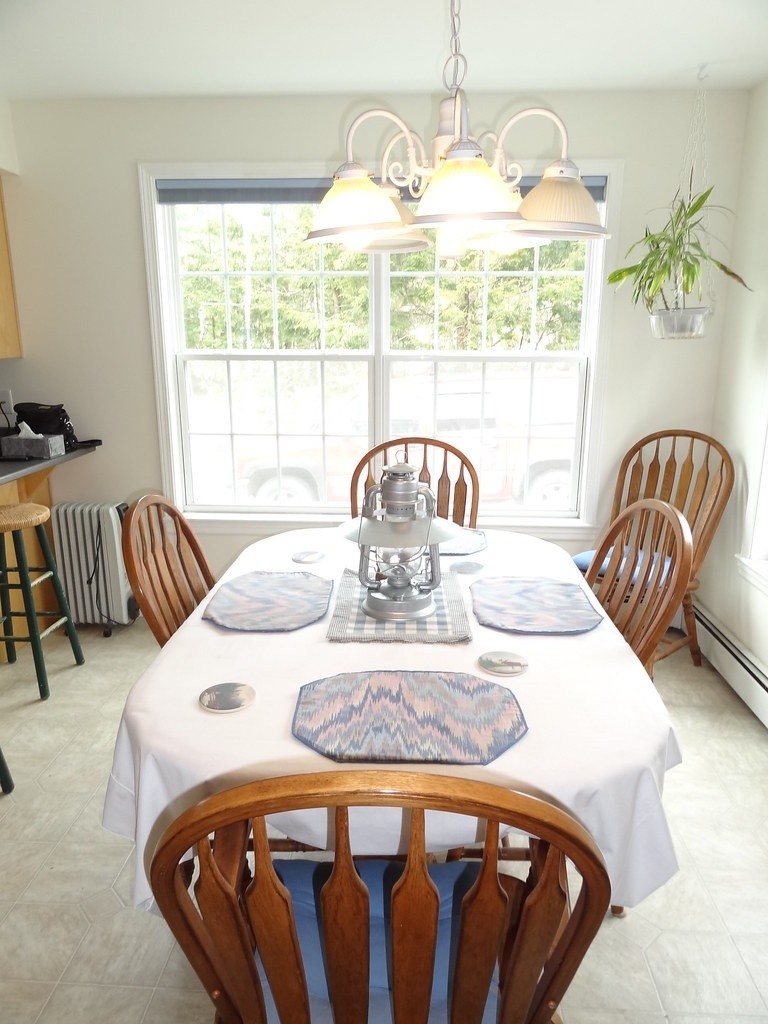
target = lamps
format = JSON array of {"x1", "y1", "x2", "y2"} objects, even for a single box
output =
[
  {"x1": 301, "y1": 0, "x2": 612, "y2": 255},
  {"x1": 338, "y1": 449, "x2": 467, "y2": 623}
]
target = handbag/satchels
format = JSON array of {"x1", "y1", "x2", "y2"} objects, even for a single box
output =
[{"x1": 13, "y1": 402, "x2": 78, "y2": 451}]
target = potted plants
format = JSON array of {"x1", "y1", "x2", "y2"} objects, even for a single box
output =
[{"x1": 606, "y1": 163, "x2": 753, "y2": 339}]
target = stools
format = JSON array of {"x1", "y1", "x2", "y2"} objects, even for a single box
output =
[{"x1": 0, "y1": 502, "x2": 85, "y2": 700}]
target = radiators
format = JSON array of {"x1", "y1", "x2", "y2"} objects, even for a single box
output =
[{"x1": 51, "y1": 501, "x2": 140, "y2": 637}]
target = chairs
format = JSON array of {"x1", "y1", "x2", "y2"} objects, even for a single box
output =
[
  {"x1": 350, "y1": 439, "x2": 479, "y2": 528},
  {"x1": 121, "y1": 493, "x2": 217, "y2": 648},
  {"x1": 571, "y1": 430, "x2": 735, "y2": 684},
  {"x1": 583, "y1": 499, "x2": 692, "y2": 667},
  {"x1": 149, "y1": 772, "x2": 611, "y2": 1024}
]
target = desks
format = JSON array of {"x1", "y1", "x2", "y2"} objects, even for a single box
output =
[
  {"x1": 103, "y1": 526, "x2": 682, "y2": 917},
  {"x1": 1, "y1": 446, "x2": 98, "y2": 660}
]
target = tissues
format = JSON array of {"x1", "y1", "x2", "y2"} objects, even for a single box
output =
[{"x1": 0, "y1": 420, "x2": 67, "y2": 460}]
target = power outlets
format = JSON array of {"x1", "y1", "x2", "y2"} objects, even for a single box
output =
[{"x1": 0, "y1": 389, "x2": 14, "y2": 414}]
